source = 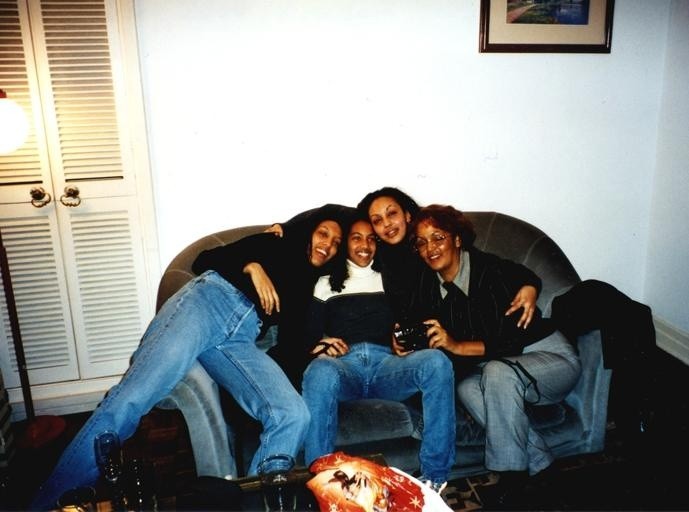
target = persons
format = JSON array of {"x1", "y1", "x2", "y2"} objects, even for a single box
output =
[
  {"x1": 295, "y1": 220, "x2": 459, "y2": 498},
  {"x1": 22, "y1": 213, "x2": 351, "y2": 510},
  {"x1": 261, "y1": 185, "x2": 545, "y2": 413},
  {"x1": 388, "y1": 202, "x2": 584, "y2": 512}
]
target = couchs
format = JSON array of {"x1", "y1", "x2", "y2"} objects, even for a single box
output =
[{"x1": 128, "y1": 202, "x2": 612, "y2": 481}]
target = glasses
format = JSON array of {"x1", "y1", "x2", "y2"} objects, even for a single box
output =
[{"x1": 412, "y1": 234, "x2": 450, "y2": 252}]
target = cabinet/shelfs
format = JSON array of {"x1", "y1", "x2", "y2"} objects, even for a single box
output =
[{"x1": 0, "y1": 0, "x2": 162, "y2": 421}]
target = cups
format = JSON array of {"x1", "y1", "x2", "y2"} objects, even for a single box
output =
[
  {"x1": 56, "y1": 486, "x2": 97, "y2": 512},
  {"x1": 256, "y1": 453, "x2": 299, "y2": 511},
  {"x1": 95, "y1": 428, "x2": 126, "y2": 483}
]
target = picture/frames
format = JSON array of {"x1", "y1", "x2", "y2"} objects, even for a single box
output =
[{"x1": 478, "y1": 0, "x2": 618, "y2": 55}]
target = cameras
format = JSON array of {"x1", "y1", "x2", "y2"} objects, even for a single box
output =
[{"x1": 392, "y1": 319, "x2": 430, "y2": 352}]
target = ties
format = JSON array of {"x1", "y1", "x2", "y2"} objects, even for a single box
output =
[{"x1": 441, "y1": 280, "x2": 460, "y2": 338}]
